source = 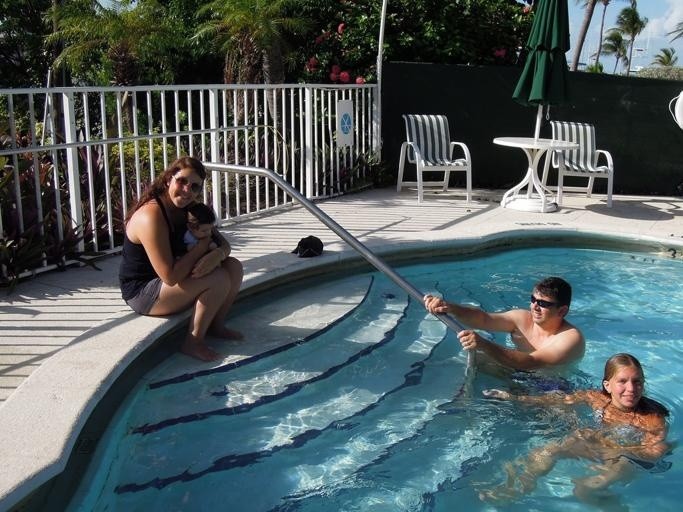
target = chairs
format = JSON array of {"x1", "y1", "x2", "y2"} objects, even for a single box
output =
[
  {"x1": 541, "y1": 119, "x2": 615, "y2": 207},
  {"x1": 398, "y1": 111, "x2": 473, "y2": 206}
]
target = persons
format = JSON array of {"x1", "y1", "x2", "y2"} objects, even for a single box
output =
[
  {"x1": 118, "y1": 158, "x2": 244, "y2": 361},
  {"x1": 182, "y1": 206, "x2": 218, "y2": 265},
  {"x1": 480, "y1": 353, "x2": 669, "y2": 502},
  {"x1": 421, "y1": 277, "x2": 586, "y2": 371}
]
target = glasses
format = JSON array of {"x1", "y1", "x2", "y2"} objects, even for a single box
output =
[
  {"x1": 530, "y1": 294, "x2": 557, "y2": 308},
  {"x1": 171, "y1": 175, "x2": 201, "y2": 194}
]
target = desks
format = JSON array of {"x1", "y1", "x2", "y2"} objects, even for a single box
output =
[{"x1": 491, "y1": 136, "x2": 579, "y2": 214}]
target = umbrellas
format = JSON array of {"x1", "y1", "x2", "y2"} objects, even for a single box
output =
[{"x1": 512, "y1": 0, "x2": 570, "y2": 141}]
